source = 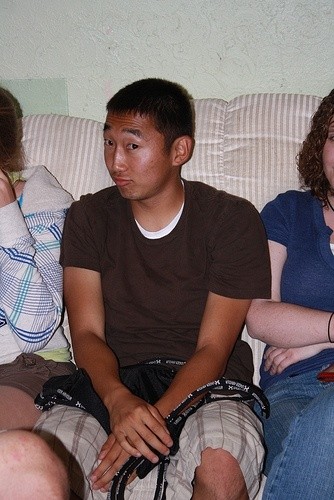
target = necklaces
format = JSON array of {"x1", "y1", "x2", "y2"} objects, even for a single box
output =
[{"x1": 326, "y1": 194, "x2": 334, "y2": 212}]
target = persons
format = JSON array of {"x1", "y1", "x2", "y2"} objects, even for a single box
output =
[
  {"x1": 244, "y1": 89, "x2": 334, "y2": 500},
  {"x1": 0, "y1": 89, "x2": 75, "y2": 432},
  {"x1": 30, "y1": 79, "x2": 272, "y2": 500},
  {"x1": 0, "y1": 429, "x2": 70, "y2": 500}
]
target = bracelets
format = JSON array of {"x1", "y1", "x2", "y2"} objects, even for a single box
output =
[{"x1": 328, "y1": 312, "x2": 334, "y2": 343}]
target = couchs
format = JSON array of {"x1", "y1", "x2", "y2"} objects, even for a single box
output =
[{"x1": 23, "y1": 94, "x2": 325, "y2": 389}]
